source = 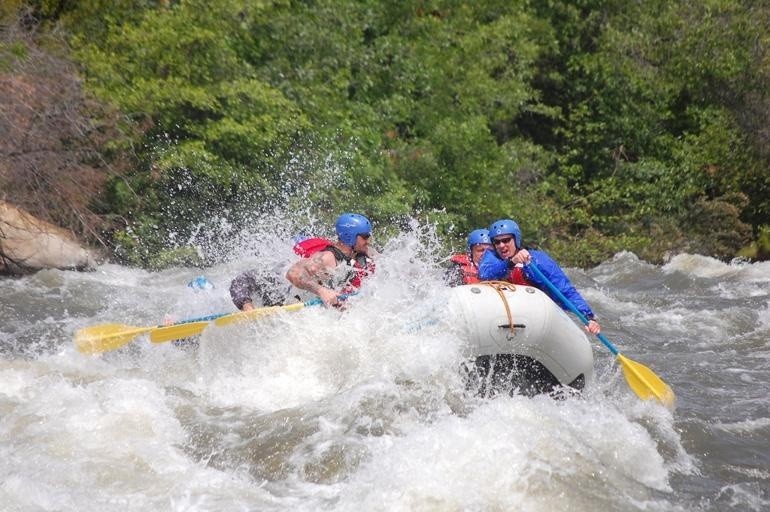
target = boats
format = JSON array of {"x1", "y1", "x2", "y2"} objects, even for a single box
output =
[{"x1": 57, "y1": 281, "x2": 595, "y2": 408}]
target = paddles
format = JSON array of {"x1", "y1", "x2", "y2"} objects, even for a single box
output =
[
  {"x1": 76, "y1": 313, "x2": 230, "y2": 356},
  {"x1": 216, "y1": 291, "x2": 356, "y2": 327},
  {"x1": 150, "y1": 322, "x2": 205, "y2": 345},
  {"x1": 527, "y1": 258, "x2": 677, "y2": 408}
]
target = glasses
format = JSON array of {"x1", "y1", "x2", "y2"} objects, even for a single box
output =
[
  {"x1": 360, "y1": 233, "x2": 370, "y2": 240},
  {"x1": 493, "y1": 236, "x2": 514, "y2": 245}
]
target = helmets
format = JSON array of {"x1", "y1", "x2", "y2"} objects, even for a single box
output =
[
  {"x1": 336, "y1": 213, "x2": 372, "y2": 250},
  {"x1": 490, "y1": 219, "x2": 521, "y2": 249},
  {"x1": 466, "y1": 228, "x2": 493, "y2": 262}
]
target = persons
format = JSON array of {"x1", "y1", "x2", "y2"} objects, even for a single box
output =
[
  {"x1": 441, "y1": 228, "x2": 494, "y2": 288},
  {"x1": 228, "y1": 267, "x2": 289, "y2": 312},
  {"x1": 478, "y1": 219, "x2": 601, "y2": 338},
  {"x1": 284, "y1": 212, "x2": 375, "y2": 312},
  {"x1": 161, "y1": 278, "x2": 216, "y2": 326}
]
